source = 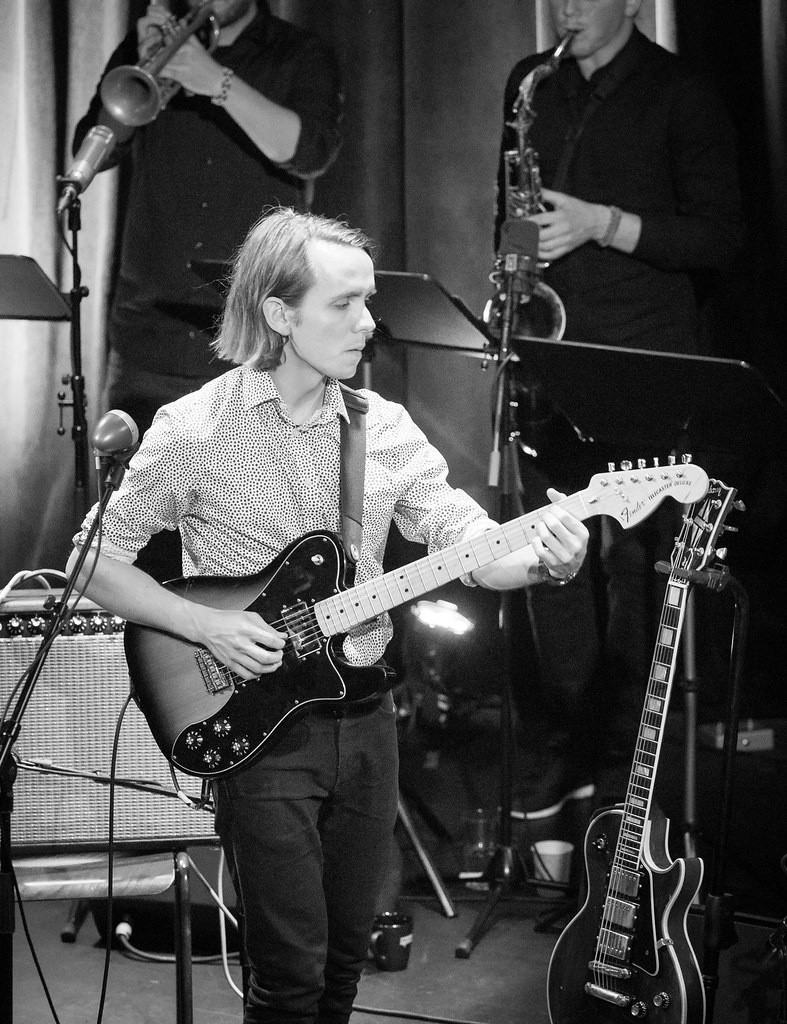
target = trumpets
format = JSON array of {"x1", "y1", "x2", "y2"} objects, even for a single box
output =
[{"x1": 97, "y1": 0, "x2": 222, "y2": 130}]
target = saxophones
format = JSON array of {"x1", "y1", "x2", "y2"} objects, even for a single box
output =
[{"x1": 478, "y1": 29, "x2": 581, "y2": 342}]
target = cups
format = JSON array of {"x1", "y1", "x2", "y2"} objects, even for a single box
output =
[
  {"x1": 530, "y1": 840, "x2": 574, "y2": 897},
  {"x1": 458, "y1": 807, "x2": 503, "y2": 892}
]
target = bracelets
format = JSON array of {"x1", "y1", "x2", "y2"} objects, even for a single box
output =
[
  {"x1": 212, "y1": 68, "x2": 233, "y2": 106},
  {"x1": 538, "y1": 548, "x2": 587, "y2": 586},
  {"x1": 598, "y1": 206, "x2": 621, "y2": 248}
]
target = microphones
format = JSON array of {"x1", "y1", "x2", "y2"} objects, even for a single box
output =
[
  {"x1": 499, "y1": 219, "x2": 539, "y2": 331},
  {"x1": 57, "y1": 125, "x2": 116, "y2": 211},
  {"x1": 92, "y1": 410, "x2": 140, "y2": 456}
]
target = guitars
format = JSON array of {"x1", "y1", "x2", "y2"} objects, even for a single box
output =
[
  {"x1": 542, "y1": 479, "x2": 749, "y2": 1024},
  {"x1": 122, "y1": 452, "x2": 713, "y2": 786}
]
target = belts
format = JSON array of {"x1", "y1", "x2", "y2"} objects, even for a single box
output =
[{"x1": 306, "y1": 699, "x2": 385, "y2": 721}]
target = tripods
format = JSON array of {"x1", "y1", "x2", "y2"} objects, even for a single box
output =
[{"x1": 411, "y1": 276, "x2": 581, "y2": 960}]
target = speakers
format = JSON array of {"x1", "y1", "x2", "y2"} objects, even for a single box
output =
[{"x1": 0, "y1": 590, "x2": 220, "y2": 857}]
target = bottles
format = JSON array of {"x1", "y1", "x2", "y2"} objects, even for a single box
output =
[{"x1": 370, "y1": 913, "x2": 413, "y2": 972}]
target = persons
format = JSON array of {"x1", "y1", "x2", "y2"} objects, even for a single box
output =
[
  {"x1": 65, "y1": 208, "x2": 590, "y2": 1024},
  {"x1": 494, "y1": 0, "x2": 742, "y2": 818},
  {"x1": 72, "y1": 1, "x2": 345, "y2": 584}
]
